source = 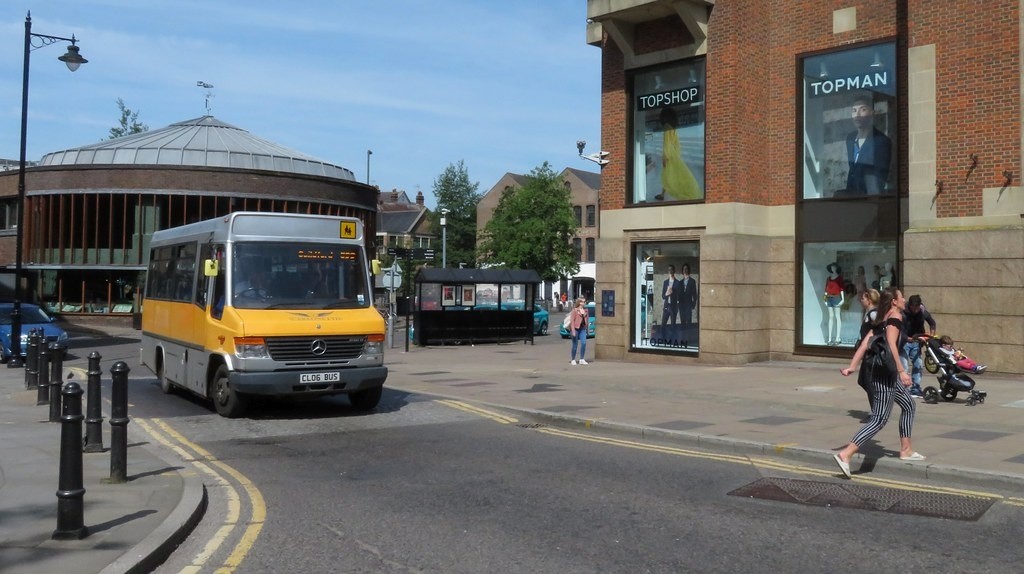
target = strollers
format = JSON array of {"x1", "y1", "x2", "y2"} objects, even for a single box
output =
[{"x1": 910, "y1": 333, "x2": 988, "y2": 408}]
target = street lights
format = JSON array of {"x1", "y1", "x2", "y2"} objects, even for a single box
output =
[
  {"x1": 7, "y1": 9, "x2": 89, "y2": 367},
  {"x1": 439, "y1": 208, "x2": 451, "y2": 270},
  {"x1": 365, "y1": 149, "x2": 372, "y2": 185}
]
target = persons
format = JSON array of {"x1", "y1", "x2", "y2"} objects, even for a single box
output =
[
  {"x1": 845, "y1": 263, "x2": 896, "y2": 313},
  {"x1": 658, "y1": 265, "x2": 679, "y2": 344},
  {"x1": 860, "y1": 289, "x2": 881, "y2": 340},
  {"x1": 570, "y1": 297, "x2": 588, "y2": 365},
  {"x1": 676, "y1": 263, "x2": 697, "y2": 346},
  {"x1": 833, "y1": 95, "x2": 891, "y2": 195},
  {"x1": 235, "y1": 261, "x2": 282, "y2": 309},
  {"x1": 833, "y1": 286, "x2": 926, "y2": 479},
  {"x1": 654, "y1": 107, "x2": 703, "y2": 200},
  {"x1": 939, "y1": 335, "x2": 987, "y2": 374},
  {"x1": 561, "y1": 292, "x2": 567, "y2": 310},
  {"x1": 823, "y1": 262, "x2": 844, "y2": 346},
  {"x1": 555, "y1": 293, "x2": 559, "y2": 306},
  {"x1": 900, "y1": 294, "x2": 936, "y2": 398}
]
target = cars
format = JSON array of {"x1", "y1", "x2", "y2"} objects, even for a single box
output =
[
  {"x1": 465, "y1": 302, "x2": 550, "y2": 336},
  {"x1": 558, "y1": 301, "x2": 595, "y2": 338},
  {"x1": 0, "y1": 302, "x2": 70, "y2": 363}
]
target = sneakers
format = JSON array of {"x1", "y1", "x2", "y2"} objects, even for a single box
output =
[
  {"x1": 911, "y1": 387, "x2": 923, "y2": 399},
  {"x1": 571, "y1": 360, "x2": 578, "y2": 366},
  {"x1": 579, "y1": 359, "x2": 589, "y2": 366}
]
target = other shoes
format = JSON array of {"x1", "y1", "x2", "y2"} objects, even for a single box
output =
[{"x1": 976, "y1": 365, "x2": 988, "y2": 373}]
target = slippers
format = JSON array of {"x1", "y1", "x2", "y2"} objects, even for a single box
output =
[
  {"x1": 833, "y1": 454, "x2": 851, "y2": 479},
  {"x1": 900, "y1": 451, "x2": 926, "y2": 461}
]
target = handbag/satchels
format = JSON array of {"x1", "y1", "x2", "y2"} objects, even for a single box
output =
[{"x1": 866, "y1": 334, "x2": 900, "y2": 373}]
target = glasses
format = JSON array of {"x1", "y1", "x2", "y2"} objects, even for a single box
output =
[{"x1": 580, "y1": 300, "x2": 587, "y2": 303}]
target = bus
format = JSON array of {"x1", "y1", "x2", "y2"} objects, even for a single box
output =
[{"x1": 138, "y1": 211, "x2": 389, "y2": 419}]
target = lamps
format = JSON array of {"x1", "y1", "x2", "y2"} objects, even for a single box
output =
[
  {"x1": 820, "y1": 63, "x2": 830, "y2": 78},
  {"x1": 868, "y1": 52, "x2": 883, "y2": 69},
  {"x1": 690, "y1": 68, "x2": 697, "y2": 84},
  {"x1": 577, "y1": 140, "x2": 612, "y2": 166},
  {"x1": 654, "y1": 76, "x2": 664, "y2": 90},
  {"x1": 645, "y1": 246, "x2": 662, "y2": 257},
  {"x1": 692, "y1": 244, "x2": 699, "y2": 257}
]
